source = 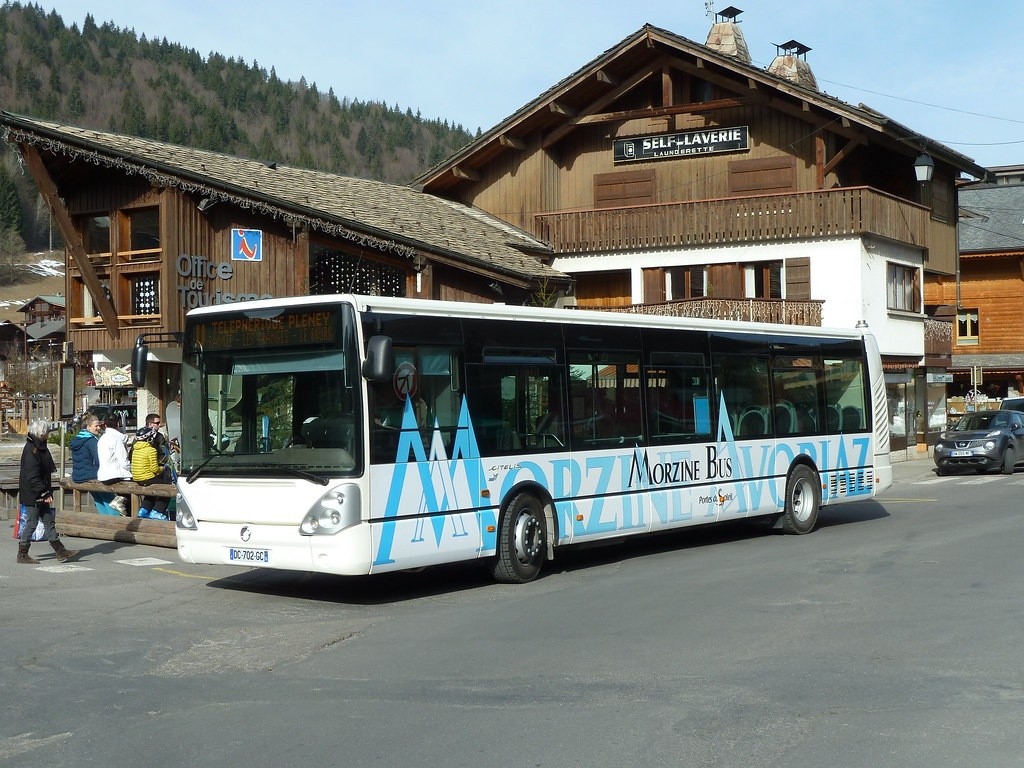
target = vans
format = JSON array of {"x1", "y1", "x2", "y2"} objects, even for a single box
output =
[{"x1": 994, "y1": 395, "x2": 1024, "y2": 429}]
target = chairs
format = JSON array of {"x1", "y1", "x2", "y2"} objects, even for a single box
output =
[
  {"x1": 301, "y1": 413, "x2": 355, "y2": 455},
  {"x1": 728, "y1": 398, "x2": 864, "y2": 436}
]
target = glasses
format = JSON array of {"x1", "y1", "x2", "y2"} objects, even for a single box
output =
[{"x1": 151, "y1": 421, "x2": 160, "y2": 425}]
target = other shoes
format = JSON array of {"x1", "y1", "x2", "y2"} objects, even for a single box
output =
[{"x1": 109, "y1": 496, "x2": 128, "y2": 515}]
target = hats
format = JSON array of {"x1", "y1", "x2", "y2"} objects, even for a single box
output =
[{"x1": 136, "y1": 426, "x2": 155, "y2": 442}]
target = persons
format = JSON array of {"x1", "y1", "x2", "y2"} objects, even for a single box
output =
[
  {"x1": 69, "y1": 412, "x2": 178, "y2": 521},
  {"x1": 376, "y1": 397, "x2": 430, "y2": 449},
  {"x1": 17, "y1": 421, "x2": 80, "y2": 564}
]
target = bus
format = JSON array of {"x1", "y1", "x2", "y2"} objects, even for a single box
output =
[{"x1": 131, "y1": 294, "x2": 894, "y2": 586}]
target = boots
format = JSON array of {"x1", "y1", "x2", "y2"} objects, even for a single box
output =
[
  {"x1": 50, "y1": 540, "x2": 79, "y2": 562},
  {"x1": 17, "y1": 541, "x2": 39, "y2": 564}
]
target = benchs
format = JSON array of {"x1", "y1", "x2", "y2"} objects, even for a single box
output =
[{"x1": 60, "y1": 477, "x2": 177, "y2": 518}]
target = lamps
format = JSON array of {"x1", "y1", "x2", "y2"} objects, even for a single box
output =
[
  {"x1": 912, "y1": 147, "x2": 934, "y2": 187},
  {"x1": 198, "y1": 197, "x2": 219, "y2": 211},
  {"x1": 489, "y1": 280, "x2": 503, "y2": 294}
]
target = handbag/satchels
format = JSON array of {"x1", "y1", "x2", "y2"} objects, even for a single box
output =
[{"x1": 12, "y1": 503, "x2": 56, "y2": 542}]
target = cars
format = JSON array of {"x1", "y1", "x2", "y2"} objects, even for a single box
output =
[{"x1": 933, "y1": 410, "x2": 1024, "y2": 475}]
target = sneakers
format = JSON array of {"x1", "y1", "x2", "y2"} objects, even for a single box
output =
[
  {"x1": 138, "y1": 507, "x2": 150, "y2": 518},
  {"x1": 148, "y1": 509, "x2": 166, "y2": 520}
]
